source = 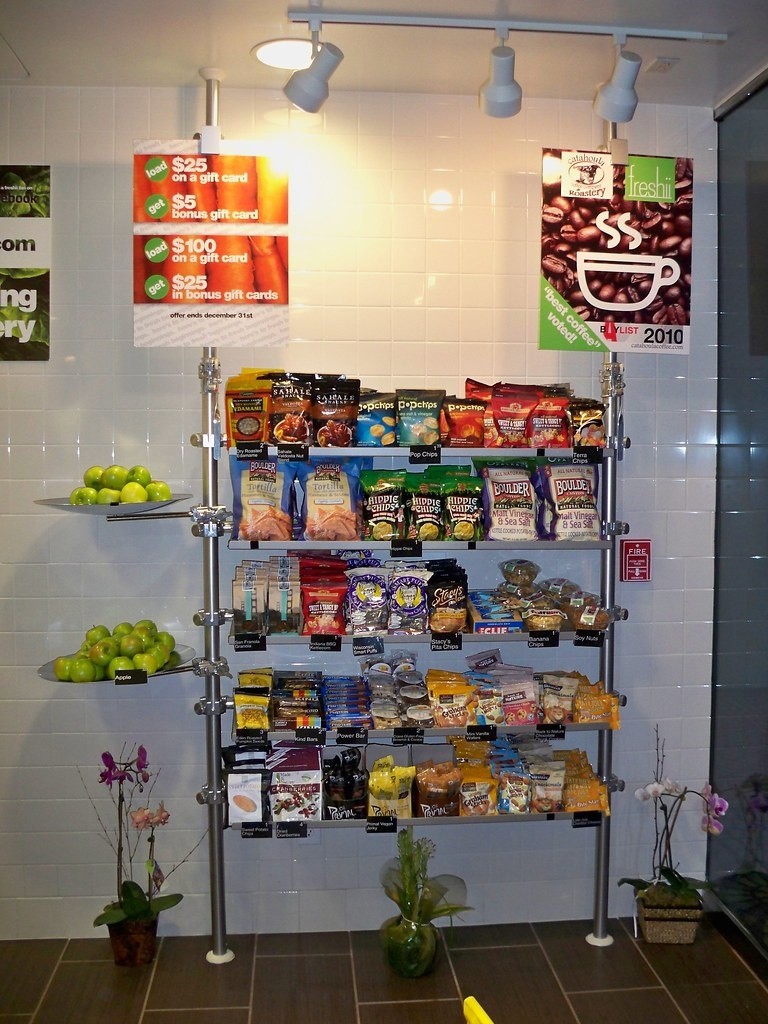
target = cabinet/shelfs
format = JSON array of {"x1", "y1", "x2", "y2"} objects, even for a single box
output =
[{"x1": 35, "y1": 68, "x2": 631, "y2": 965}]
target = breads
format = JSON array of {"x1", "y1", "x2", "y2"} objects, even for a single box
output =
[{"x1": 497, "y1": 560, "x2": 610, "y2": 629}]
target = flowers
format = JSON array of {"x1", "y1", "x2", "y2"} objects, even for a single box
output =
[
  {"x1": 379, "y1": 825, "x2": 475, "y2": 938},
  {"x1": 618, "y1": 724, "x2": 729, "y2": 906},
  {"x1": 734, "y1": 772, "x2": 768, "y2": 871},
  {"x1": 77, "y1": 740, "x2": 209, "y2": 928}
]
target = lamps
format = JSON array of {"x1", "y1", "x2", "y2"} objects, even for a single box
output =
[
  {"x1": 593, "y1": 50, "x2": 642, "y2": 122},
  {"x1": 478, "y1": 46, "x2": 522, "y2": 118},
  {"x1": 282, "y1": 43, "x2": 344, "y2": 113}
]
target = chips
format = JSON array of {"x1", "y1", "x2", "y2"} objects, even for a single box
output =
[
  {"x1": 454, "y1": 521, "x2": 474, "y2": 540},
  {"x1": 419, "y1": 523, "x2": 439, "y2": 541},
  {"x1": 372, "y1": 522, "x2": 392, "y2": 541}
]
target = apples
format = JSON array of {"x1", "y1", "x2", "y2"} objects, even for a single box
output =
[
  {"x1": 54, "y1": 619, "x2": 175, "y2": 682},
  {"x1": 70, "y1": 466, "x2": 172, "y2": 505}
]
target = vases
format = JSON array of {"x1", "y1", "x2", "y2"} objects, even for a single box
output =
[
  {"x1": 380, "y1": 913, "x2": 440, "y2": 978},
  {"x1": 108, "y1": 902, "x2": 160, "y2": 965},
  {"x1": 634, "y1": 889, "x2": 704, "y2": 944}
]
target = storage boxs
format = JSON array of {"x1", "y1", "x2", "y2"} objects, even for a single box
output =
[
  {"x1": 467, "y1": 589, "x2": 523, "y2": 634},
  {"x1": 411, "y1": 743, "x2": 459, "y2": 817},
  {"x1": 321, "y1": 745, "x2": 367, "y2": 820},
  {"x1": 365, "y1": 743, "x2": 412, "y2": 819}
]
